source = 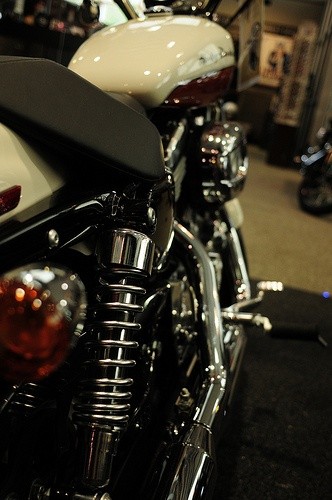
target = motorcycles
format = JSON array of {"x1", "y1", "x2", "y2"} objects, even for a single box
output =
[
  {"x1": 4, "y1": 0, "x2": 284, "y2": 499},
  {"x1": 298, "y1": 116, "x2": 332, "y2": 216}
]
